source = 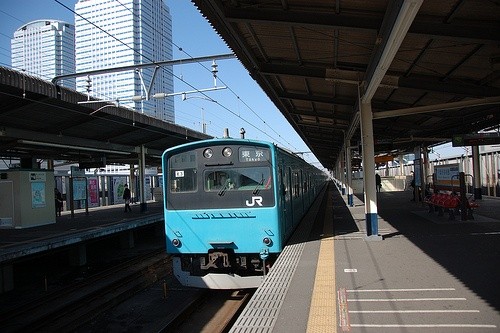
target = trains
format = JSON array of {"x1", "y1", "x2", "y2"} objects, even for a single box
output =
[{"x1": 161, "y1": 138, "x2": 330, "y2": 290}]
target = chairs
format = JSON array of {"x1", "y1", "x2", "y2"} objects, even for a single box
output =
[{"x1": 423, "y1": 193, "x2": 480, "y2": 221}]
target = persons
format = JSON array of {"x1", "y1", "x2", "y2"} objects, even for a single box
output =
[
  {"x1": 54, "y1": 188, "x2": 63, "y2": 216},
  {"x1": 411, "y1": 172, "x2": 422, "y2": 202},
  {"x1": 123, "y1": 184, "x2": 131, "y2": 213},
  {"x1": 376, "y1": 174, "x2": 382, "y2": 192}
]
